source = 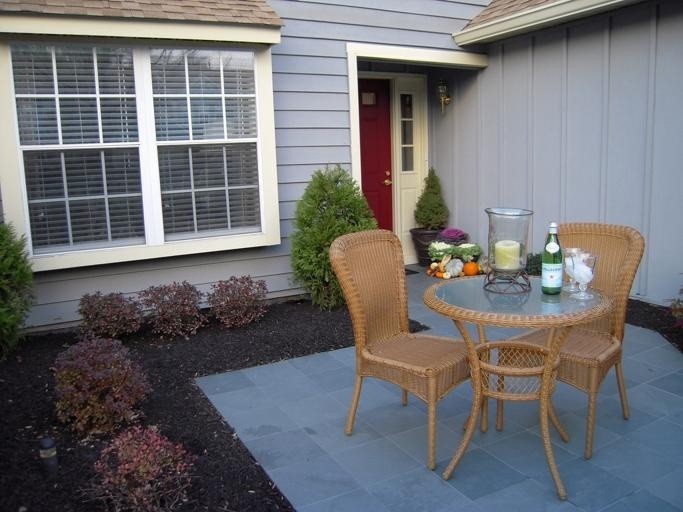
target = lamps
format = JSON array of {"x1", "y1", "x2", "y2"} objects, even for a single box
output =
[{"x1": 434, "y1": 77, "x2": 450, "y2": 113}]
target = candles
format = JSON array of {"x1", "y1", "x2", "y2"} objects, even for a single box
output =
[{"x1": 495, "y1": 240, "x2": 520, "y2": 269}]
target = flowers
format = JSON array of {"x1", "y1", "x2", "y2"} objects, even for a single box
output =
[{"x1": 429, "y1": 229, "x2": 483, "y2": 262}]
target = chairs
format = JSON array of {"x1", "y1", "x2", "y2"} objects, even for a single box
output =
[
  {"x1": 328, "y1": 229, "x2": 486, "y2": 469},
  {"x1": 496, "y1": 222, "x2": 646, "y2": 459}
]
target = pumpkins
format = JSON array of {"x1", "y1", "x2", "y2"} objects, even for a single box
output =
[{"x1": 427, "y1": 258, "x2": 479, "y2": 279}]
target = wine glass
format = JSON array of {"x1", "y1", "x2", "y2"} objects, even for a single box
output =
[{"x1": 563, "y1": 248, "x2": 597, "y2": 301}]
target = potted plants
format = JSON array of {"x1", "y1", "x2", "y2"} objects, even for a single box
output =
[{"x1": 410, "y1": 165, "x2": 449, "y2": 267}]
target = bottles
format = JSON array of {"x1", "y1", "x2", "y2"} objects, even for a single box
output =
[{"x1": 541, "y1": 223, "x2": 562, "y2": 295}]
